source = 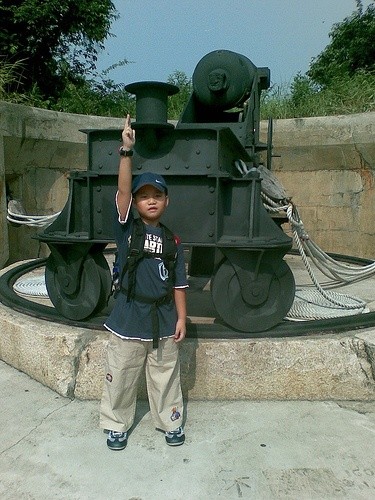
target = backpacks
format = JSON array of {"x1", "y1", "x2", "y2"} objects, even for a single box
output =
[{"x1": 111, "y1": 217, "x2": 178, "y2": 298}]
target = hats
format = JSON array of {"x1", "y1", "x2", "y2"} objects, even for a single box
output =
[{"x1": 132, "y1": 172, "x2": 168, "y2": 195}]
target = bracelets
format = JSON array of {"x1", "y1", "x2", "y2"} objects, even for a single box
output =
[{"x1": 118, "y1": 146, "x2": 133, "y2": 156}]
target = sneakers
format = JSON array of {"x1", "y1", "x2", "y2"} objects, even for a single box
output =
[
  {"x1": 165, "y1": 426, "x2": 185, "y2": 446},
  {"x1": 107, "y1": 430, "x2": 127, "y2": 449}
]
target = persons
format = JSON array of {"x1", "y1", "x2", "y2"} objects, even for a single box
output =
[{"x1": 99, "y1": 114, "x2": 189, "y2": 450}]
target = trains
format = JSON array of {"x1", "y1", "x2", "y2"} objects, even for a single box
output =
[{"x1": 30, "y1": 49, "x2": 296, "y2": 332}]
